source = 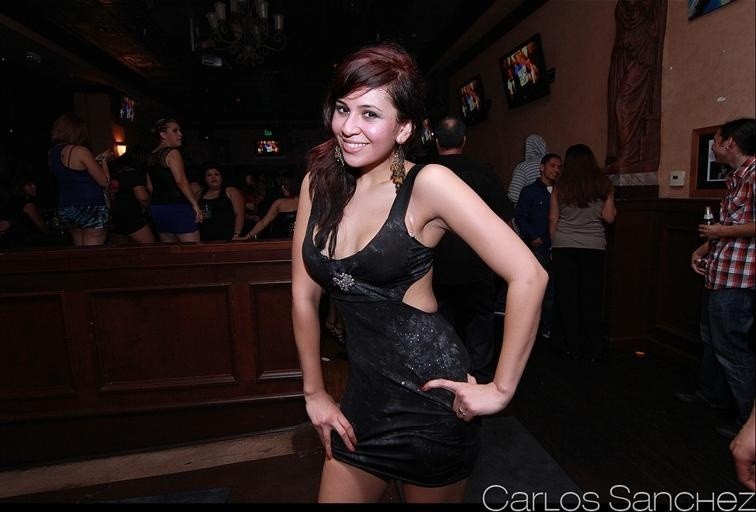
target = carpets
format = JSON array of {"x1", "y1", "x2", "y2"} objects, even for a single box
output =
[{"x1": 393, "y1": 410, "x2": 589, "y2": 502}]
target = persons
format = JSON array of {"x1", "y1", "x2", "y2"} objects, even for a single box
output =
[
  {"x1": 514, "y1": 152, "x2": 563, "y2": 340},
  {"x1": 454, "y1": 49, "x2": 541, "y2": 124},
  {"x1": 729, "y1": 398, "x2": 756, "y2": 492},
  {"x1": 548, "y1": 143, "x2": 620, "y2": 367},
  {"x1": 287, "y1": 42, "x2": 551, "y2": 502},
  {"x1": 672, "y1": 118, "x2": 755, "y2": 439}
]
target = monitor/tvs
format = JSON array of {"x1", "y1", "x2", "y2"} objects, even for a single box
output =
[
  {"x1": 454, "y1": 76, "x2": 489, "y2": 126},
  {"x1": 499, "y1": 33, "x2": 550, "y2": 109},
  {"x1": 255, "y1": 136, "x2": 284, "y2": 156},
  {"x1": 112, "y1": 92, "x2": 136, "y2": 126}
]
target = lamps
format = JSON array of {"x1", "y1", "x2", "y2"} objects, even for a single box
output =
[{"x1": 182, "y1": 0, "x2": 290, "y2": 73}]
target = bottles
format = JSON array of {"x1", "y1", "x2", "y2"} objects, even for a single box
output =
[{"x1": 703, "y1": 205, "x2": 715, "y2": 227}]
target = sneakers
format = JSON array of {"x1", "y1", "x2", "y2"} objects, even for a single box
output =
[
  {"x1": 715, "y1": 421, "x2": 742, "y2": 439},
  {"x1": 674, "y1": 389, "x2": 725, "y2": 410}
]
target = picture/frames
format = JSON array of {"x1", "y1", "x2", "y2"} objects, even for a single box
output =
[{"x1": 689, "y1": 122, "x2": 736, "y2": 200}]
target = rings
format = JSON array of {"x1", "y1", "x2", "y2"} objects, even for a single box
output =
[{"x1": 457, "y1": 407, "x2": 467, "y2": 419}]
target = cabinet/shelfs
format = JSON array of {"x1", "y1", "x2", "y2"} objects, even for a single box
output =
[{"x1": 594, "y1": 195, "x2": 714, "y2": 385}]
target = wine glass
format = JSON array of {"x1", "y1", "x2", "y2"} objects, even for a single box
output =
[{"x1": 199, "y1": 203, "x2": 210, "y2": 222}]
